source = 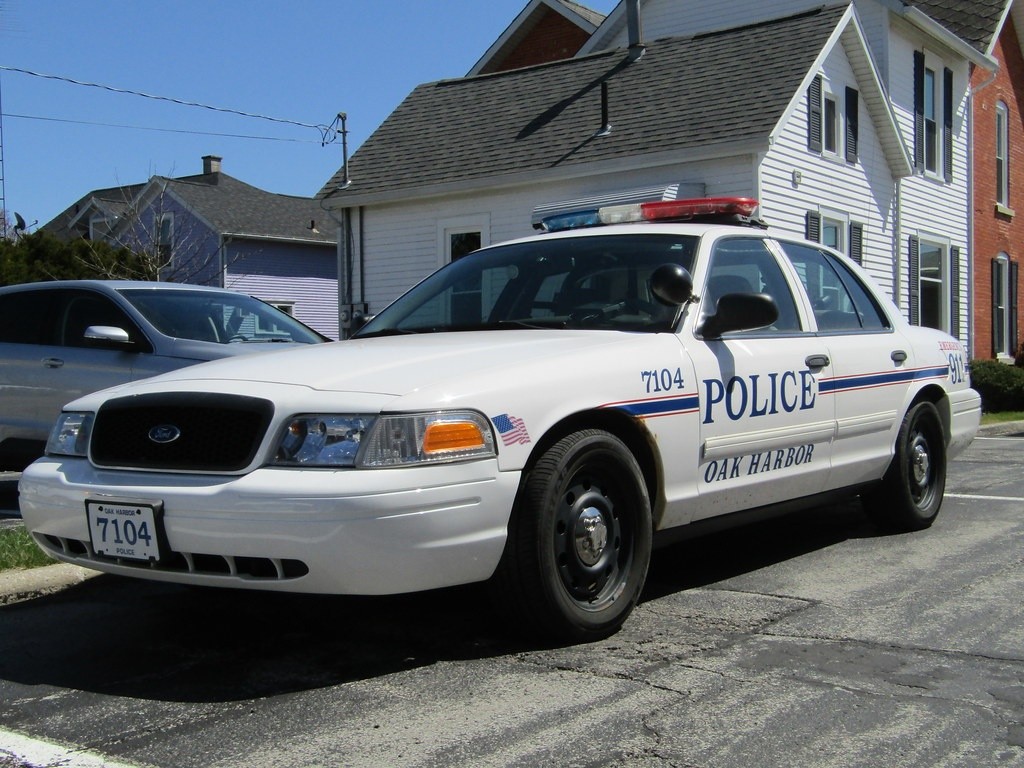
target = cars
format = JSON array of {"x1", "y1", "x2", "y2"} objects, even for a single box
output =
[
  {"x1": 18, "y1": 193, "x2": 983, "y2": 642},
  {"x1": 1, "y1": 277, "x2": 337, "y2": 506}
]
